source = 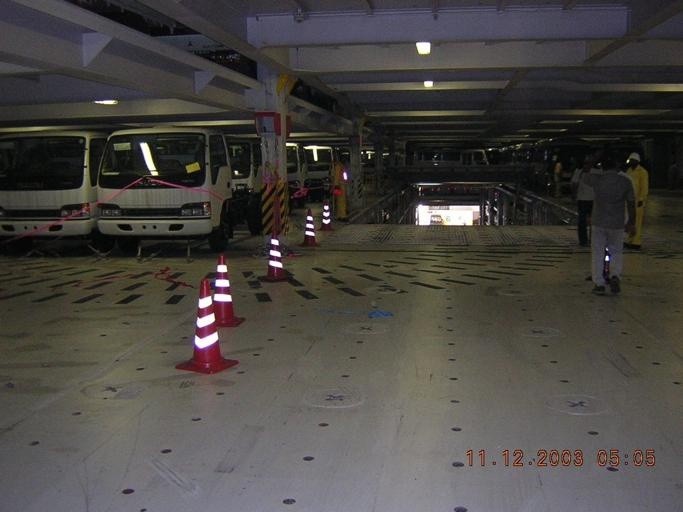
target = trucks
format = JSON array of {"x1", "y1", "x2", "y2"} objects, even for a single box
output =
[{"x1": 369, "y1": 151, "x2": 596, "y2": 193}]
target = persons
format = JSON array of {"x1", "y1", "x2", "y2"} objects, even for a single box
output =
[
  {"x1": 623, "y1": 151, "x2": 649, "y2": 249},
  {"x1": 571, "y1": 153, "x2": 596, "y2": 245},
  {"x1": 545, "y1": 160, "x2": 561, "y2": 197},
  {"x1": 582, "y1": 153, "x2": 636, "y2": 295}
]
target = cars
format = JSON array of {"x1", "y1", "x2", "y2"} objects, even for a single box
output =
[{"x1": 429, "y1": 214, "x2": 442, "y2": 226}]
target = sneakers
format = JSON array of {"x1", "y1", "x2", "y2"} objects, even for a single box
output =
[
  {"x1": 623, "y1": 242, "x2": 641, "y2": 252},
  {"x1": 592, "y1": 276, "x2": 621, "y2": 294}
]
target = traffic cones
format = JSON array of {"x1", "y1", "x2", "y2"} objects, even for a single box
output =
[
  {"x1": 168, "y1": 276, "x2": 238, "y2": 373},
  {"x1": 213, "y1": 254, "x2": 243, "y2": 330},
  {"x1": 267, "y1": 229, "x2": 284, "y2": 281},
  {"x1": 303, "y1": 208, "x2": 316, "y2": 245},
  {"x1": 320, "y1": 200, "x2": 331, "y2": 230}
]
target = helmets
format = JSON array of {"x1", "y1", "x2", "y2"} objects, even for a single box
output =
[{"x1": 629, "y1": 152, "x2": 640, "y2": 162}]
